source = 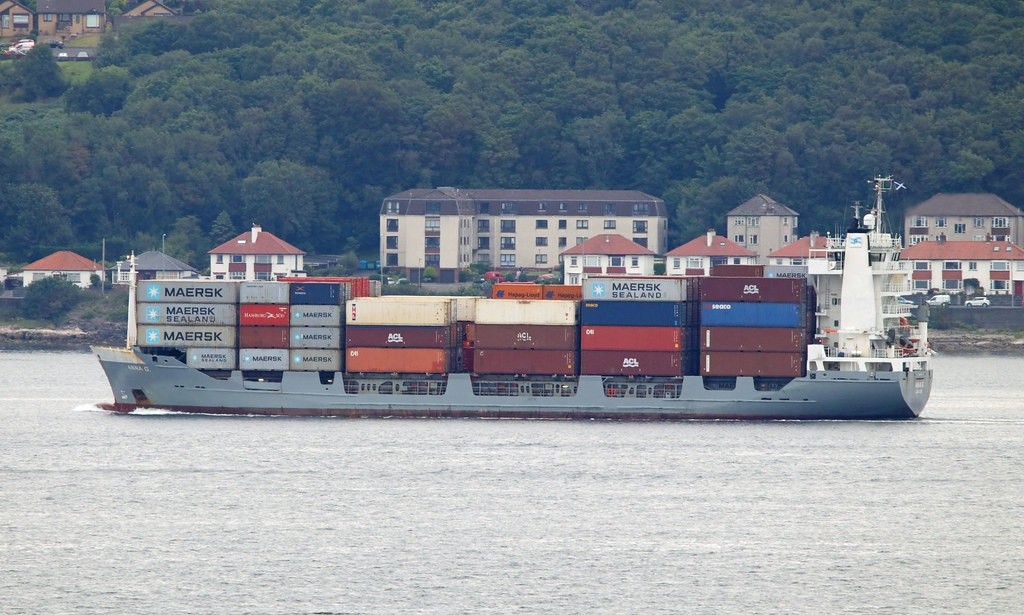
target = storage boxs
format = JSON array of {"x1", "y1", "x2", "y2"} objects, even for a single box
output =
[{"x1": 135, "y1": 262, "x2": 818, "y2": 375}]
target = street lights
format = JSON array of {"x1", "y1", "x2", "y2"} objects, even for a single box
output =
[{"x1": 162, "y1": 233, "x2": 167, "y2": 279}]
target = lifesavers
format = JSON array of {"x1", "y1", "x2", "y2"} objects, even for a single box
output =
[{"x1": 608, "y1": 388, "x2": 616, "y2": 396}]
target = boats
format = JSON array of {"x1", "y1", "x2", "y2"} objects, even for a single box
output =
[{"x1": 88, "y1": 173, "x2": 936, "y2": 424}]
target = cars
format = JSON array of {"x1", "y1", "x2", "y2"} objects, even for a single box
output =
[
  {"x1": 964, "y1": 297, "x2": 991, "y2": 307},
  {"x1": 897, "y1": 297, "x2": 915, "y2": 305},
  {"x1": 386, "y1": 276, "x2": 407, "y2": 285}
]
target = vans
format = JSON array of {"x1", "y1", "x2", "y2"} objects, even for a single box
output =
[
  {"x1": 926, "y1": 294, "x2": 951, "y2": 308},
  {"x1": 485, "y1": 270, "x2": 506, "y2": 283}
]
